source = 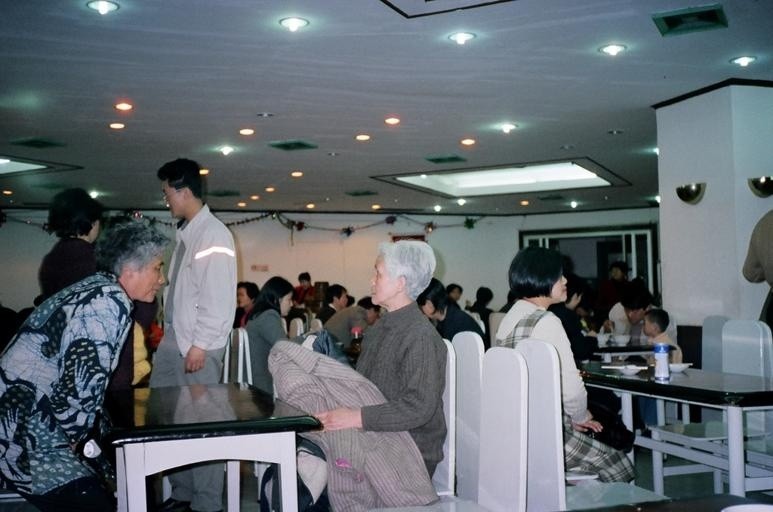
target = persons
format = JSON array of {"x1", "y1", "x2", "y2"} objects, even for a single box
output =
[
  {"x1": 346, "y1": 295, "x2": 354, "y2": 308},
  {"x1": 601, "y1": 286, "x2": 676, "y2": 349},
  {"x1": 638, "y1": 310, "x2": 683, "y2": 440},
  {"x1": 0, "y1": 217, "x2": 166, "y2": 512},
  {"x1": 418, "y1": 278, "x2": 488, "y2": 354},
  {"x1": 233, "y1": 277, "x2": 293, "y2": 394},
  {"x1": 446, "y1": 285, "x2": 462, "y2": 303},
  {"x1": 260, "y1": 242, "x2": 441, "y2": 512},
  {"x1": 322, "y1": 296, "x2": 381, "y2": 350},
  {"x1": 315, "y1": 285, "x2": 348, "y2": 327},
  {"x1": 39, "y1": 188, "x2": 134, "y2": 430},
  {"x1": 572, "y1": 293, "x2": 595, "y2": 334},
  {"x1": 498, "y1": 246, "x2": 639, "y2": 487},
  {"x1": 234, "y1": 282, "x2": 263, "y2": 333},
  {"x1": 154, "y1": 158, "x2": 239, "y2": 512},
  {"x1": 173, "y1": 384, "x2": 234, "y2": 426},
  {"x1": 286, "y1": 290, "x2": 307, "y2": 340},
  {"x1": 466, "y1": 287, "x2": 496, "y2": 351},
  {"x1": 600, "y1": 262, "x2": 629, "y2": 318},
  {"x1": 548, "y1": 272, "x2": 598, "y2": 364},
  {"x1": 743, "y1": 210, "x2": 773, "y2": 335},
  {"x1": 292, "y1": 273, "x2": 316, "y2": 311}
]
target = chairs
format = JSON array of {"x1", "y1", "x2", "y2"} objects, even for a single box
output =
[
  {"x1": 453, "y1": 331, "x2": 601, "y2": 498},
  {"x1": 759, "y1": 321, "x2": 773, "y2": 437},
  {"x1": 516, "y1": 337, "x2": 669, "y2": 512},
  {"x1": 369, "y1": 346, "x2": 528, "y2": 512},
  {"x1": 241, "y1": 325, "x2": 272, "y2": 503},
  {"x1": 430, "y1": 338, "x2": 455, "y2": 499},
  {"x1": 701, "y1": 317, "x2": 725, "y2": 422},
  {"x1": 648, "y1": 319, "x2": 764, "y2": 497},
  {"x1": 221, "y1": 329, "x2": 243, "y2": 511}
]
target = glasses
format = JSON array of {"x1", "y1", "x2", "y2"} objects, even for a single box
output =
[{"x1": 162, "y1": 188, "x2": 182, "y2": 203}]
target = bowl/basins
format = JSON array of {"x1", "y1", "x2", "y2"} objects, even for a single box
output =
[
  {"x1": 598, "y1": 334, "x2": 608, "y2": 343},
  {"x1": 619, "y1": 369, "x2": 648, "y2": 374},
  {"x1": 615, "y1": 335, "x2": 630, "y2": 347},
  {"x1": 671, "y1": 362, "x2": 691, "y2": 377}
]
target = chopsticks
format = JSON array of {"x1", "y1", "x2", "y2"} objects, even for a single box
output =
[{"x1": 601, "y1": 365, "x2": 648, "y2": 370}]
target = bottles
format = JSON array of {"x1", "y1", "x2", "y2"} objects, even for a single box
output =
[{"x1": 654, "y1": 342, "x2": 671, "y2": 382}]
